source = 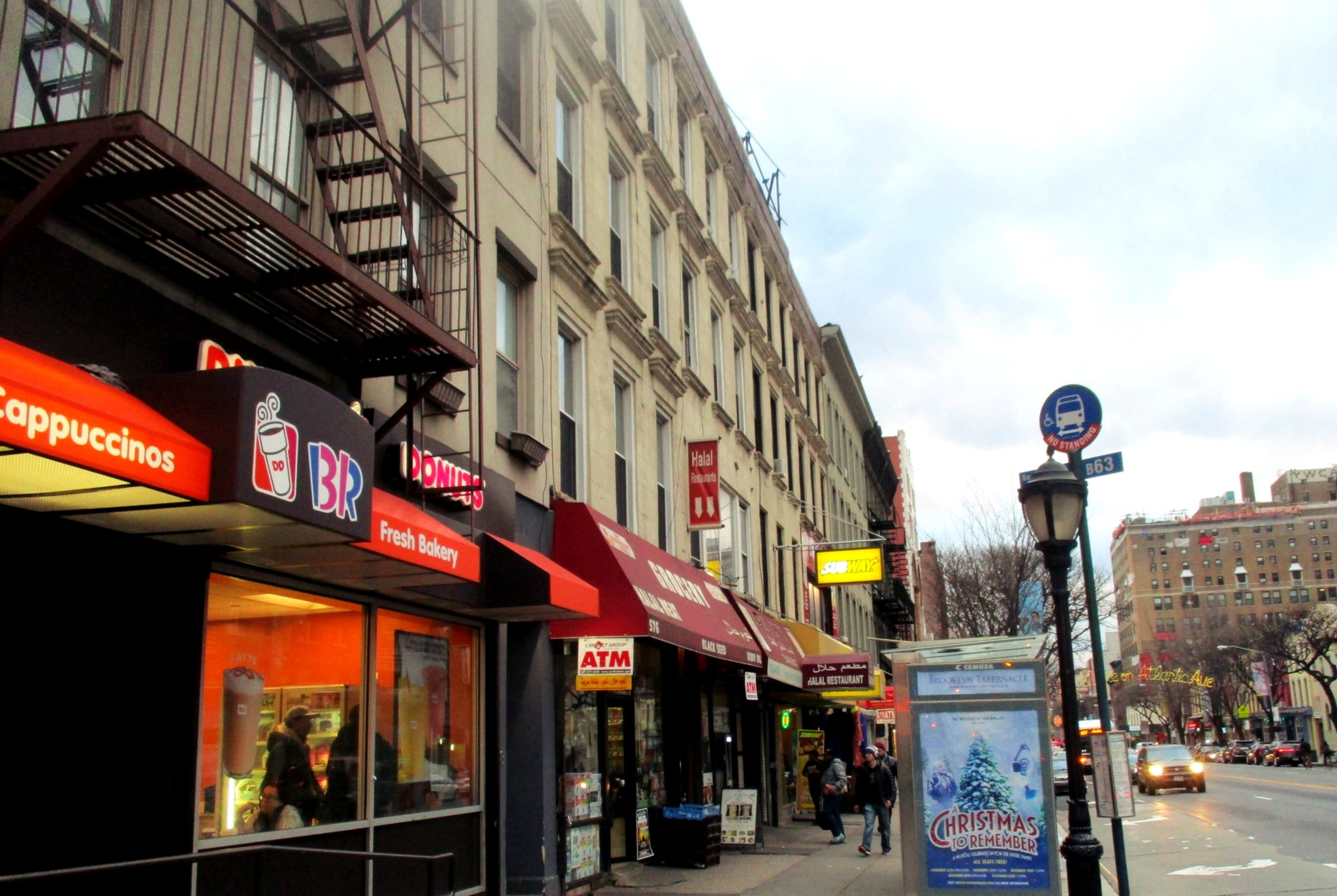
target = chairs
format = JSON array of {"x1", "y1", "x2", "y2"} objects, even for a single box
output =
[{"x1": 285, "y1": 780, "x2": 435, "y2": 830}]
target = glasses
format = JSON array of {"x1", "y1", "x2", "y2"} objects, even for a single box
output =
[{"x1": 260, "y1": 794, "x2": 279, "y2": 801}]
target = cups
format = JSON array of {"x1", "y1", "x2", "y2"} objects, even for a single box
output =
[
  {"x1": 420, "y1": 665, "x2": 447, "y2": 747},
  {"x1": 397, "y1": 681, "x2": 429, "y2": 783},
  {"x1": 221, "y1": 677, "x2": 262, "y2": 780}
]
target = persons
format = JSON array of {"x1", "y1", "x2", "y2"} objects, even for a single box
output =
[
  {"x1": 852, "y1": 746, "x2": 896, "y2": 855},
  {"x1": 320, "y1": 705, "x2": 397, "y2": 819},
  {"x1": 244, "y1": 786, "x2": 304, "y2": 833},
  {"x1": 565, "y1": 748, "x2": 584, "y2": 776},
  {"x1": 874, "y1": 741, "x2": 898, "y2": 833},
  {"x1": 818, "y1": 748, "x2": 848, "y2": 842},
  {"x1": 258, "y1": 705, "x2": 323, "y2": 828},
  {"x1": 801, "y1": 750, "x2": 826, "y2": 826}
]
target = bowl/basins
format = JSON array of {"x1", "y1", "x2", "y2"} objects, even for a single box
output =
[{"x1": 202, "y1": 831, "x2": 215, "y2": 839}]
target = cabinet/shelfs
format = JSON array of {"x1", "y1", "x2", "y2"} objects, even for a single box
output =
[{"x1": 568, "y1": 700, "x2": 640, "y2": 774}]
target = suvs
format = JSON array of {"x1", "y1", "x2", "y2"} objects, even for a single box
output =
[
  {"x1": 1220, "y1": 740, "x2": 1253, "y2": 764},
  {"x1": 1263, "y1": 740, "x2": 1303, "y2": 767},
  {"x1": 1245, "y1": 742, "x2": 1273, "y2": 766}
]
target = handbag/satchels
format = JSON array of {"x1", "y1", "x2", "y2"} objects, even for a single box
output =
[{"x1": 823, "y1": 783, "x2": 843, "y2": 796}]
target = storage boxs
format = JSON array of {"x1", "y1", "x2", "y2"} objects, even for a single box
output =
[{"x1": 659, "y1": 802, "x2": 723, "y2": 868}]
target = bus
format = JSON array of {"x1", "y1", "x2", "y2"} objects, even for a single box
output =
[{"x1": 1079, "y1": 719, "x2": 1117, "y2": 775}]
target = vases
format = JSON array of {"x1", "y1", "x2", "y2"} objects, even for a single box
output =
[
  {"x1": 510, "y1": 431, "x2": 548, "y2": 463},
  {"x1": 422, "y1": 372, "x2": 466, "y2": 412}
]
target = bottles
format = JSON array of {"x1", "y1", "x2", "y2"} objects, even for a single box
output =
[
  {"x1": 580, "y1": 857, "x2": 593, "y2": 869},
  {"x1": 580, "y1": 836, "x2": 598, "y2": 850},
  {"x1": 582, "y1": 848, "x2": 592, "y2": 862}
]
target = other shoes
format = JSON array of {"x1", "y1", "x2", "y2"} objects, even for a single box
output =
[
  {"x1": 858, "y1": 845, "x2": 871, "y2": 856},
  {"x1": 830, "y1": 833, "x2": 845, "y2": 844},
  {"x1": 841, "y1": 838, "x2": 846, "y2": 843},
  {"x1": 882, "y1": 849, "x2": 892, "y2": 855}
]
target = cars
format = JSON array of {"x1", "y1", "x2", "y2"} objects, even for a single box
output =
[
  {"x1": 1127, "y1": 741, "x2": 1155, "y2": 780},
  {"x1": 1051, "y1": 737, "x2": 1088, "y2": 795},
  {"x1": 1134, "y1": 744, "x2": 1206, "y2": 796},
  {"x1": 1187, "y1": 742, "x2": 1221, "y2": 763},
  {"x1": 419, "y1": 770, "x2": 469, "y2": 802}
]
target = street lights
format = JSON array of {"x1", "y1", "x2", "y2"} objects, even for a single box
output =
[
  {"x1": 1216, "y1": 645, "x2": 1280, "y2": 741},
  {"x1": 1016, "y1": 444, "x2": 1106, "y2": 896},
  {"x1": 1093, "y1": 704, "x2": 1115, "y2": 724},
  {"x1": 1138, "y1": 684, "x2": 1174, "y2": 745}
]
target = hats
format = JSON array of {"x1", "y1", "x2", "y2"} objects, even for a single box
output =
[
  {"x1": 285, "y1": 703, "x2": 322, "y2": 719},
  {"x1": 874, "y1": 742, "x2": 885, "y2": 751},
  {"x1": 864, "y1": 745, "x2": 879, "y2": 759},
  {"x1": 819, "y1": 748, "x2": 836, "y2": 757}
]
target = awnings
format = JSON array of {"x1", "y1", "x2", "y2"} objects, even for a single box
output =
[
  {"x1": 780, "y1": 620, "x2": 888, "y2": 702},
  {"x1": 725, "y1": 591, "x2": 802, "y2": 691},
  {"x1": 548, "y1": 502, "x2": 764, "y2": 671}
]
